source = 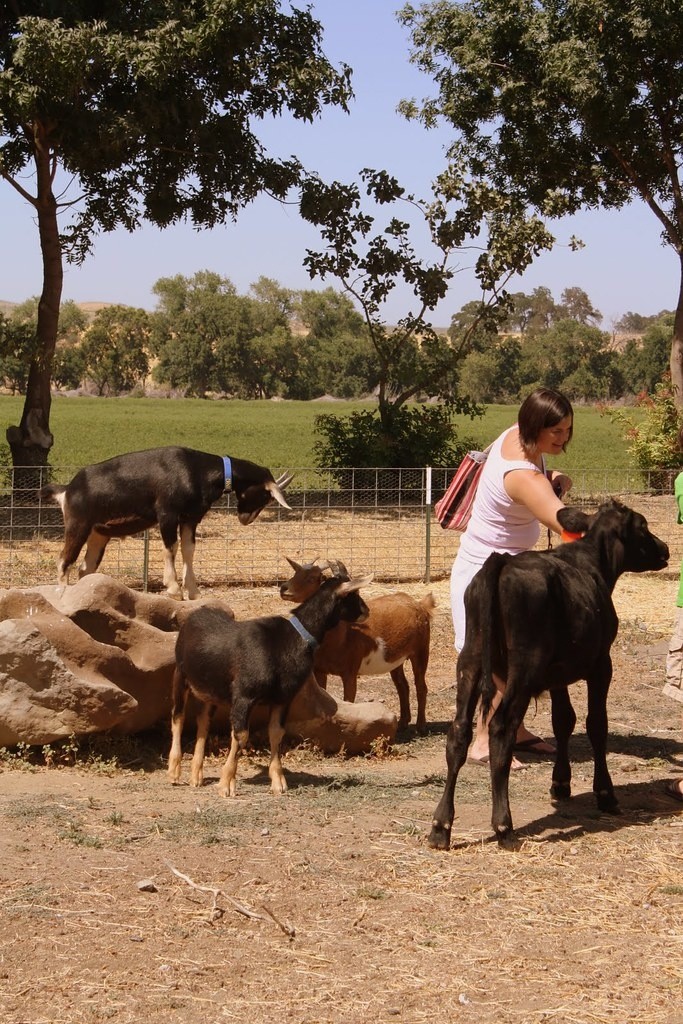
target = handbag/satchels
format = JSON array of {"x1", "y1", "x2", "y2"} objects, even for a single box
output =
[{"x1": 434, "y1": 441, "x2": 496, "y2": 531}]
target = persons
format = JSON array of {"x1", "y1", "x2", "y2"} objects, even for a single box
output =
[
  {"x1": 663, "y1": 472, "x2": 683, "y2": 802},
  {"x1": 450, "y1": 387, "x2": 586, "y2": 765}
]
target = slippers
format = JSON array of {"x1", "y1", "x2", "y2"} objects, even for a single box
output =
[
  {"x1": 515, "y1": 737, "x2": 555, "y2": 754},
  {"x1": 467, "y1": 754, "x2": 490, "y2": 769},
  {"x1": 664, "y1": 777, "x2": 683, "y2": 801}
]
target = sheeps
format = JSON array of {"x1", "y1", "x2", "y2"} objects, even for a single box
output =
[
  {"x1": 279, "y1": 552, "x2": 436, "y2": 735},
  {"x1": 167, "y1": 558, "x2": 373, "y2": 798}
]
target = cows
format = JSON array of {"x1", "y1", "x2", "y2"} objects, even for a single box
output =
[
  {"x1": 36, "y1": 446, "x2": 296, "y2": 601},
  {"x1": 425, "y1": 496, "x2": 671, "y2": 851}
]
